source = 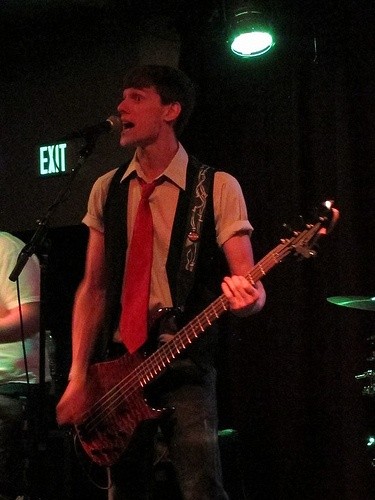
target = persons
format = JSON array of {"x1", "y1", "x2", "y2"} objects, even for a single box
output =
[
  {"x1": 55, "y1": 64, "x2": 266, "y2": 500},
  {"x1": 0, "y1": 231, "x2": 52, "y2": 500}
]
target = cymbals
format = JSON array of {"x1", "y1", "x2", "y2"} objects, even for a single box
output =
[{"x1": 325, "y1": 294, "x2": 374, "y2": 312}]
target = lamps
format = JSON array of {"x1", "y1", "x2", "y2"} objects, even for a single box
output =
[{"x1": 225, "y1": 0, "x2": 277, "y2": 58}]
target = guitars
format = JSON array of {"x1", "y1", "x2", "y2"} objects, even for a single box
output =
[{"x1": 74, "y1": 200, "x2": 341, "y2": 468}]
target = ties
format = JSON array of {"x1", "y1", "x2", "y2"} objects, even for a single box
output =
[{"x1": 119, "y1": 174, "x2": 168, "y2": 353}]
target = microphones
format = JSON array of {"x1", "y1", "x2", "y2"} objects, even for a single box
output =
[{"x1": 57, "y1": 115, "x2": 122, "y2": 145}]
